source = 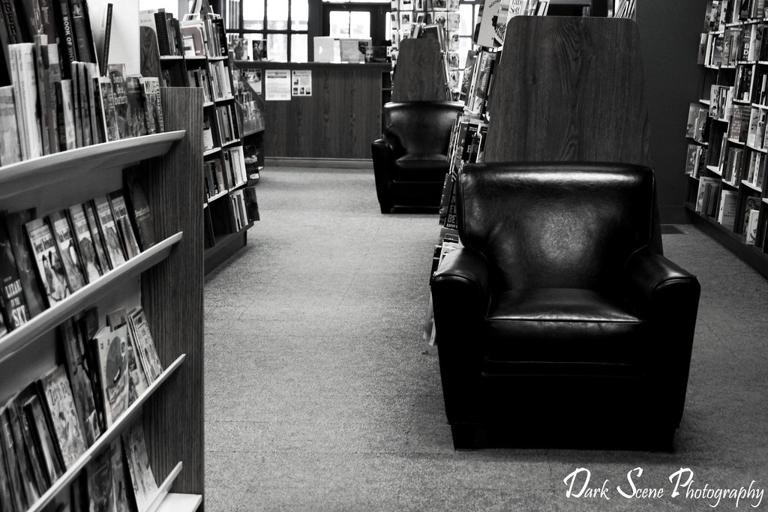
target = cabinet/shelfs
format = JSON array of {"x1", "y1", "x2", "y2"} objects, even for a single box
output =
[
  {"x1": 679, "y1": 1, "x2": 768, "y2": 280},
  {"x1": 0, "y1": 0, "x2": 267, "y2": 512}
]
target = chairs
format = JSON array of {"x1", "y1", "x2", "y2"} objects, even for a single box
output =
[
  {"x1": 371, "y1": 101, "x2": 464, "y2": 214},
  {"x1": 429, "y1": 161, "x2": 702, "y2": 453}
]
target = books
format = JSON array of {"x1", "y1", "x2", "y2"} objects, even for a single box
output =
[
  {"x1": 385, "y1": 1, "x2": 549, "y2": 344},
  {"x1": 685, "y1": 0, "x2": 768, "y2": 248},
  {"x1": 0, "y1": 3, "x2": 265, "y2": 512}
]
